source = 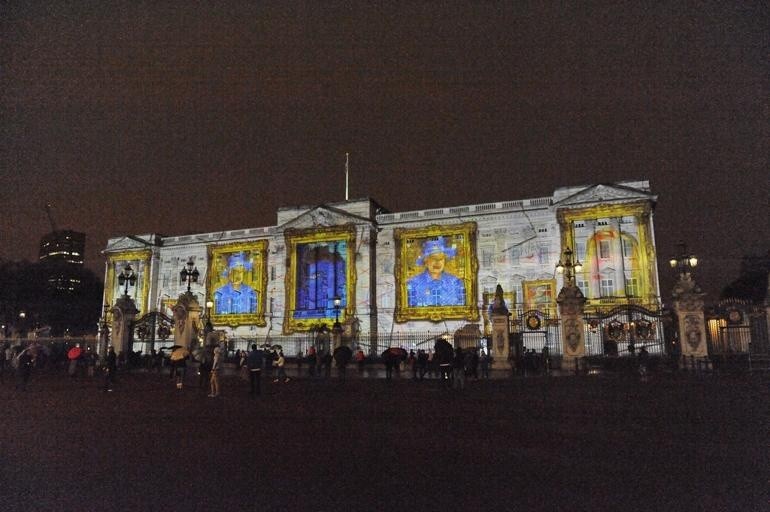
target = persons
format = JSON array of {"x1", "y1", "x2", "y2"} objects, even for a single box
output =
[
  {"x1": 406, "y1": 239, "x2": 464, "y2": 306},
  {"x1": 212, "y1": 253, "x2": 258, "y2": 317},
  {"x1": 638, "y1": 346, "x2": 649, "y2": 384}
]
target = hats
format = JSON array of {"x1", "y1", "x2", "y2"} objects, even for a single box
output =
[
  {"x1": 414, "y1": 238, "x2": 459, "y2": 268},
  {"x1": 222, "y1": 253, "x2": 255, "y2": 279}
]
